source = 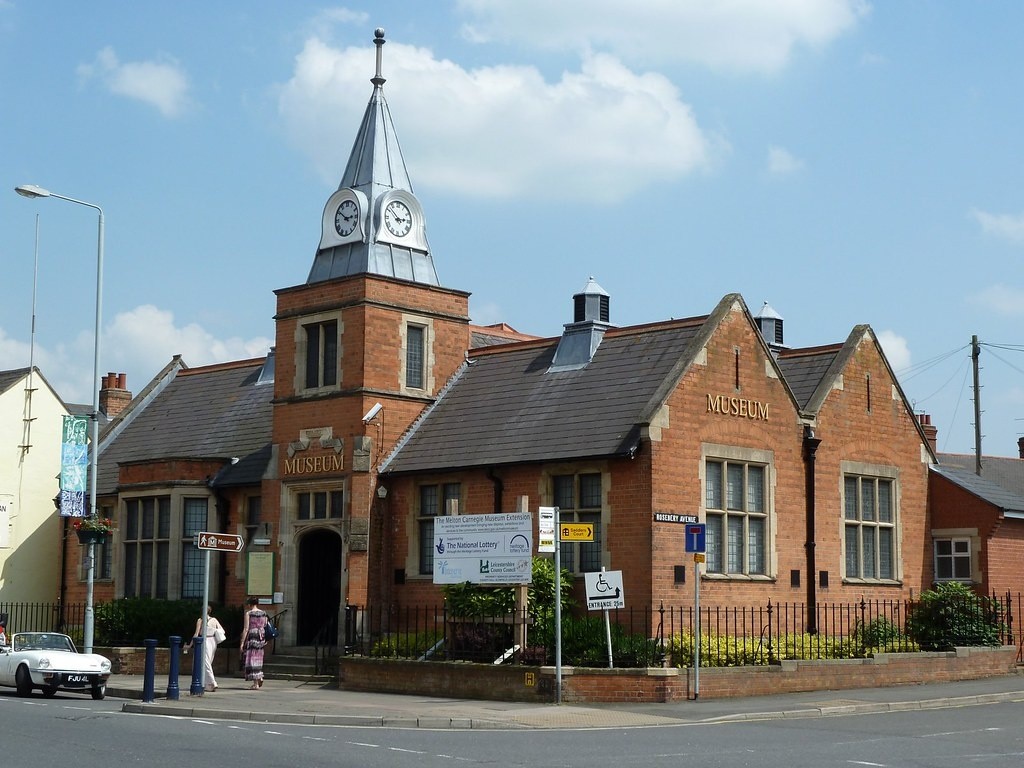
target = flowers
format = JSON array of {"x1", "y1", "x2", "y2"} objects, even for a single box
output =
[{"x1": 62, "y1": 513, "x2": 113, "y2": 537}]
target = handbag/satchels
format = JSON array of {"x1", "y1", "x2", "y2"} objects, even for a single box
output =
[
  {"x1": 214, "y1": 628, "x2": 227, "y2": 644},
  {"x1": 264, "y1": 619, "x2": 279, "y2": 640}
]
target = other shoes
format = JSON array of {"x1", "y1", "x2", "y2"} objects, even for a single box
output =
[
  {"x1": 250, "y1": 685, "x2": 259, "y2": 689},
  {"x1": 259, "y1": 678, "x2": 263, "y2": 687},
  {"x1": 210, "y1": 683, "x2": 218, "y2": 691}
]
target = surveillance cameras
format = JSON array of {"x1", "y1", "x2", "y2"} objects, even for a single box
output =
[{"x1": 362, "y1": 402, "x2": 383, "y2": 423}]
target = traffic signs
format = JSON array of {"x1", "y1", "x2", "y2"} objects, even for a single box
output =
[
  {"x1": 559, "y1": 521, "x2": 596, "y2": 543},
  {"x1": 197, "y1": 532, "x2": 245, "y2": 553}
]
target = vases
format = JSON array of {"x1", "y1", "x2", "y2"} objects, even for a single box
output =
[{"x1": 76, "y1": 530, "x2": 105, "y2": 544}]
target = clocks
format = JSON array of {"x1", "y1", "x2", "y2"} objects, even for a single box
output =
[
  {"x1": 384, "y1": 201, "x2": 412, "y2": 238},
  {"x1": 335, "y1": 200, "x2": 358, "y2": 237}
]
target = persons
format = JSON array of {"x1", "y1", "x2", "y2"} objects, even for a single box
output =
[
  {"x1": 183, "y1": 606, "x2": 225, "y2": 692},
  {"x1": 240, "y1": 596, "x2": 268, "y2": 690}
]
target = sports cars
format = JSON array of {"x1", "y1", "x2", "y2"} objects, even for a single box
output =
[{"x1": 0, "y1": 632, "x2": 113, "y2": 701}]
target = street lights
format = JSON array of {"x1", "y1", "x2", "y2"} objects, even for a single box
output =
[{"x1": 14, "y1": 182, "x2": 104, "y2": 653}]
